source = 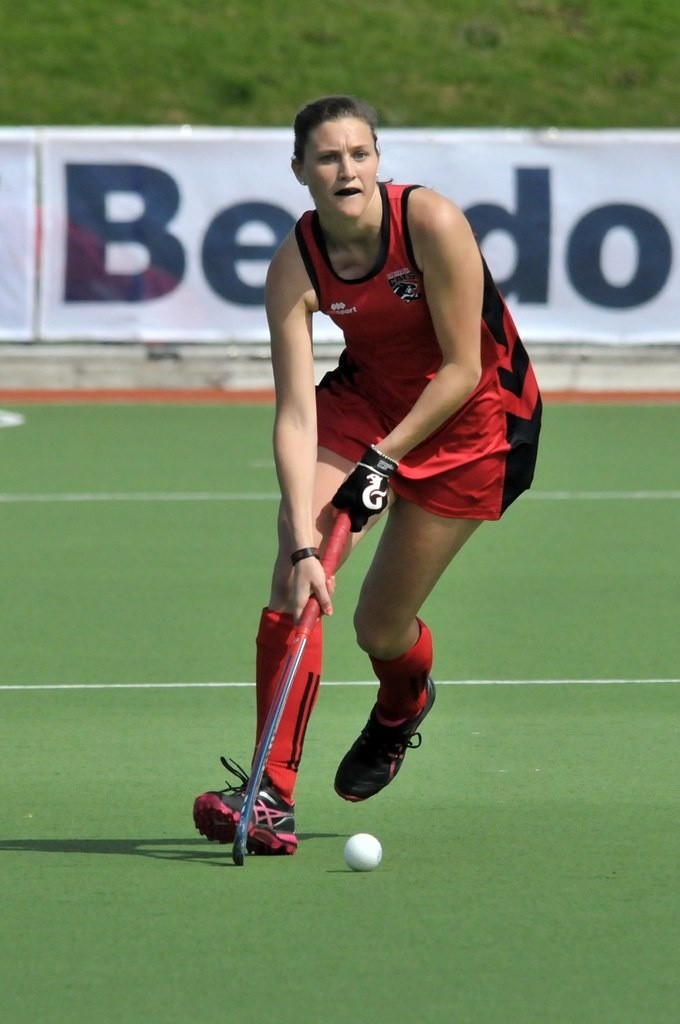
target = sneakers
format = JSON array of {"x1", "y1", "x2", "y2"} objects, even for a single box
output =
[
  {"x1": 334, "y1": 678, "x2": 436, "y2": 804},
  {"x1": 193, "y1": 756, "x2": 298, "y2": 855}
]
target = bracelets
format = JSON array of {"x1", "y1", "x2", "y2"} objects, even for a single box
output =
[{"x1": 290, "y1": 546, "x2": 321, "y2": 567}]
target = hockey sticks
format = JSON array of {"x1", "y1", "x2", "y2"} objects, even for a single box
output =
[{"x1": 230, "y1": 509, "x2": 355, "y2": 868}]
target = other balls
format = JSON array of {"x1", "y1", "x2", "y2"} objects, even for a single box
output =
[{"x1": 343, "y1": 832, "x2": 385, "y2": 872}]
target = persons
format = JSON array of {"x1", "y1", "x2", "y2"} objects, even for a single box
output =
[{"x1": 190, "y1": 97, "x2": 543, "y2": 856}]
